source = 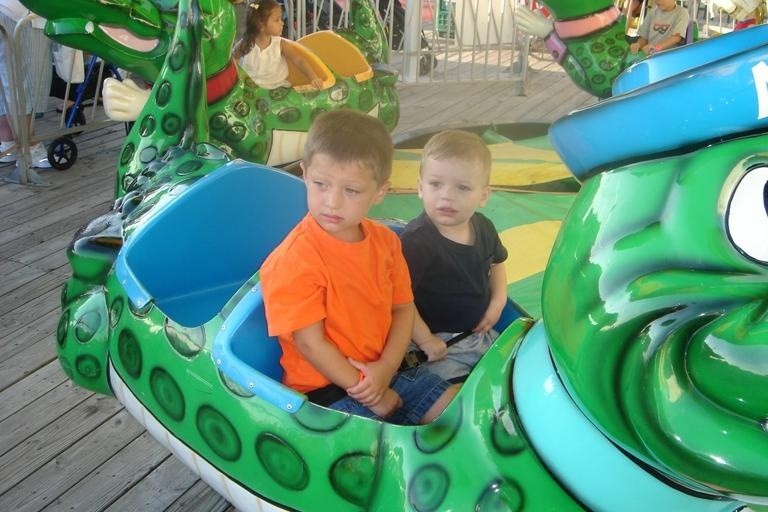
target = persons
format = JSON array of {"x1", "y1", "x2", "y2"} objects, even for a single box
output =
[
  {"x1": 0, "y1": 0, "x2": 54, "y2": 168},
  {"x1": 259, "y1": 108, "x2": 458, "y2": 425},
  {"x1": 233, "y1": 0, "x2": 323, "y2": 90},
  {"x1": 397, "y1": 129, "x2": 509, "y2": 390},
  {"x1": 630, "y1": 0, "x2": 690, "y2": 55}
]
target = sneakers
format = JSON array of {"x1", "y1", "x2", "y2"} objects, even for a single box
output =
[
  {"x1": 15, "y1": 141, "x2": 67, "y2": 168},
  {"x1": 0, "y1": 141, "x2": 17, "y2": 162}
]
